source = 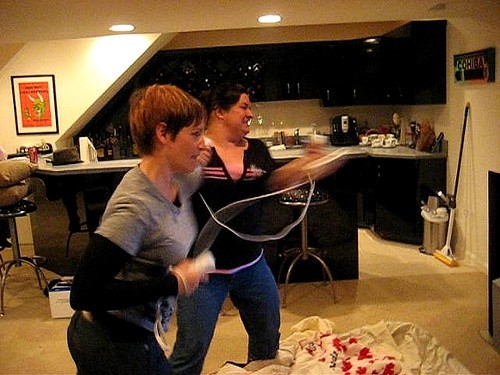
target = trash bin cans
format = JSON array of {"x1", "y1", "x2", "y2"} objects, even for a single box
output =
[{"x1": 418, "y1": 206, "x2": 450, "y2": 256}]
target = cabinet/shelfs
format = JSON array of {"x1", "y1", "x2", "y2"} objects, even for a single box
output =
[
  {"x1": 321, "y1": 38, "x2": 369, "y2": 106},
  {"x1": 203, "y1": 46, "x2": 262, "y2": 103},
  {"x1": 262, "y1": 41, "x2": 321, "y2": 101},
  {"x1": 369, "y1": 35, "x2": 411, "y2": 105},
  {"x1": 412, "y1": 19, "x2": 447, "y2": 105},
  {"x1": 336, "y1": 155, "x2": 446, "y2": 242},
  {"x1": 155, "y1": 49, "x2": 202, "y2": 100}
]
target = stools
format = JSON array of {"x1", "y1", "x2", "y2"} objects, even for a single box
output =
[
  {"x1": 277, "y1": 188, "x2": 338, "y2": 307},
  {"x1": 1, "y1": 201, "x2": 50, "y2": 315}
]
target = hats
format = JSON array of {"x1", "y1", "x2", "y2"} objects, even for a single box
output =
[{"x1": 53, "y1": 147, "x2": 84, "y2": 165}]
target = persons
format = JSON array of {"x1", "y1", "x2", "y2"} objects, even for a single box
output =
[
  {"x1": 65, "y1": 84, "x2": 217, "y2": 375},
  {"x1": 168, "y1": 80, "x2": 330, "y2": 375}
]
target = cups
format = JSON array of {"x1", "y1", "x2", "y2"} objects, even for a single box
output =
[
  {"x1": 362, "y1": 137, "x2": 368, "y2": 143},
  {"x1": 385, "y1": 139, "x2": 398, "y2": 148},
  {"x1": 369, "y1": 135, "x2": 378, "y2": 142},
  {"x1": 371, "y1": 139, "x2": 384, "y2": 147},
  {"x1": 378, "y1": 134, "x2": 386, "y2": 139},
  {"x1": 387, "y1": 134, "x2": 395, "y2": 139}
]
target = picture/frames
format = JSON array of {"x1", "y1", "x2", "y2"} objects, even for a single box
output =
[
  {"x1": 10, "y1": 75, "x2": 59, "y2": 134},
  {"x1": 453, "y1": 47, "x2": 495, "y2": 82}
]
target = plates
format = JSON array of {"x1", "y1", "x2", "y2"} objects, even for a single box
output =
[
  {"x1": 383, "y1": 146, "x2": 396, "y2": 148},
  {"x1": 307, "y1": 132, "x2": 330, "y2": 143},
  {"x1": 359, "y1": 143, "x2": 370, "y2": 146},
  {"x1": 371, "y1": 145, "x2": 383, "y2": 148},
  {"x1": 359, "y1": 142, "x2": 370, "y2": 146}
]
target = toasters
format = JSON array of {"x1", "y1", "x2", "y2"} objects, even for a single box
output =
[{"x1": 105, "y1": 139, "x2": 113, "y2": 158}]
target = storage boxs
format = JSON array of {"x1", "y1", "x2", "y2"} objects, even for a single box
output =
[{"x1": 48, "y1": 278, "x2": 76, "y2": 319}]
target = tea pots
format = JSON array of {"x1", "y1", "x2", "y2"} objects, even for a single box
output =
[{"x1": 272, "y1": 132, "x2": 287, "y2": 147}]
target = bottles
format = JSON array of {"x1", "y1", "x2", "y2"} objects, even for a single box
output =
[
  {"x1": 325, "y1": 88, "x2": 332, "y2": 107},
  {"x1": 132, "y1": 140, "x2": 139, "y2": 156},
  {"x1": 352, "y1": 88, "x2": 360, "y2": 105},
  {"x1": 107, "y1": 143, "x2": 114, "y2": 161},
  {"x1": 117, "y1": 124, "x2": 124, "y2": 144},
  {"x1": 125, "y1": 136, "x2": 134, "y2": 157},
  {"x1": 397, "y1": 86, "x2": 406, "y2": 104},
  {"x1": 286, "y1": 82, "x2": 295, "y2": 100},
  {"x1": 120, "y1": 141, "x2": 128, "y2": 157},
  {"x1": 95, "y1": 145, "x2": 106, "y2": 161},
  {"x1": 296, "y1": 81, "x2": 304, "y2": 100}
]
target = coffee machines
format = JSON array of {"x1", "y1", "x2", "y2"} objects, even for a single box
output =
[{"x1": 331, "y1": 114, "x2": 358, "y2": 145}]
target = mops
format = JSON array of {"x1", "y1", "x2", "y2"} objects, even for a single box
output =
[{"x1": 433, "y1": 105, "x2": 470, "y2": 267}]
